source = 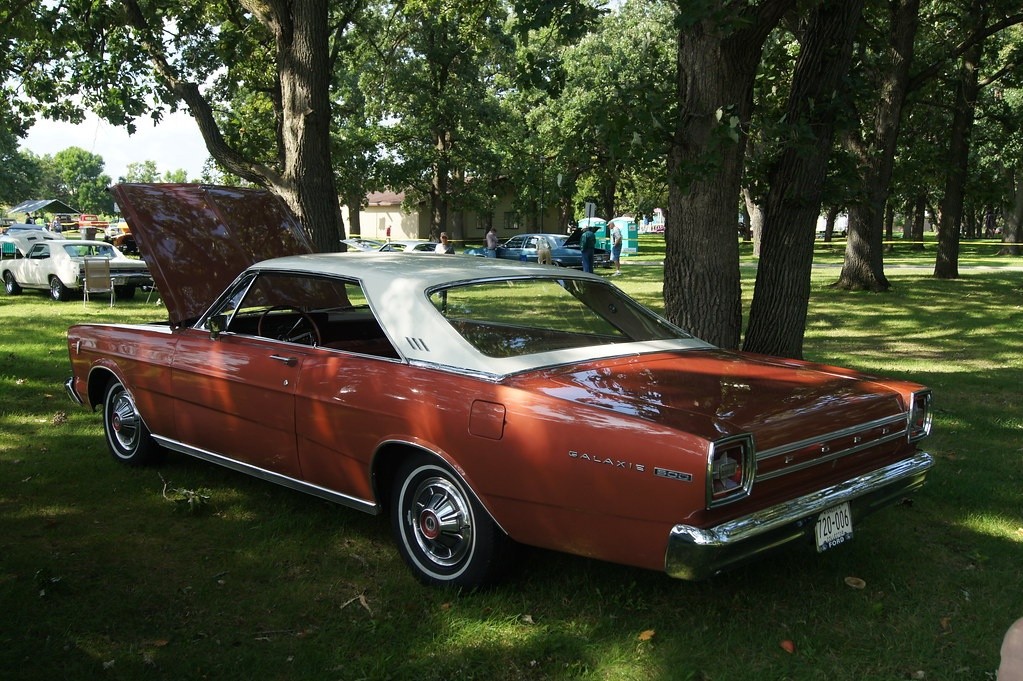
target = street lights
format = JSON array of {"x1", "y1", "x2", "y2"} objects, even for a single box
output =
[{"x1": 538, "y1": 156, "x2": 551, "y2": 234}]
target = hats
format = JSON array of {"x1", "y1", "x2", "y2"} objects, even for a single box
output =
[
  {"x1": 607, "y1": 221, "x2": 614, "y2": 225},
  {"x1": 581, "y1": 225, "x2": 588, "y2": 230},
  {"x1": 440, "y1": 232, "x2": 448, "y2": 237}
]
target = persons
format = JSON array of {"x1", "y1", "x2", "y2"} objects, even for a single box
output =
[
  {"x1": 25, "y1": 212, "x2": 32, "y2": 224},
  {"x1": 49, "y1": 217, "x2": 62, "y2": 232},
  {"x1": 606, "y1": 221, "x2": 623, "y2": 276},
  {"x1": 486, "y1": 228, "x2": 498, "y2": 258},
  {"x1": 387, "y1": 225, "x2": 391, "y2": 236},
  {"x1": 842, "y1": 230, "x2": 847, "y2": 237},
  {"x1": 580, "y1": 226, "x2": 596, "y2": 273},
  {"x1": 435, "y1": 231, "x2": 455, "y2": 254},
  {"x1": 536, "y1": 237, "x2": 551, "y2": 265}
]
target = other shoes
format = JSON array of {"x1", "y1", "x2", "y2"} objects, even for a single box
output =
[{"x1": 611, "y1": 271, "x2": 621, "y2": 276}]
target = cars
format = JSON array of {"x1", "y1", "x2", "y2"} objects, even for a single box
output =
[
  {"x1": 64, "y1": 178, "x2": 936, "y2": 598},
  {"x1": 338, "y1": 237, "x2": 438, "y2": 253},
  {"x1": 497, "y1": 225, "x2": 602, "y2": 270},
  {"x1": 0, "y1": 206, "x2": 153, "y2": 303}
]
